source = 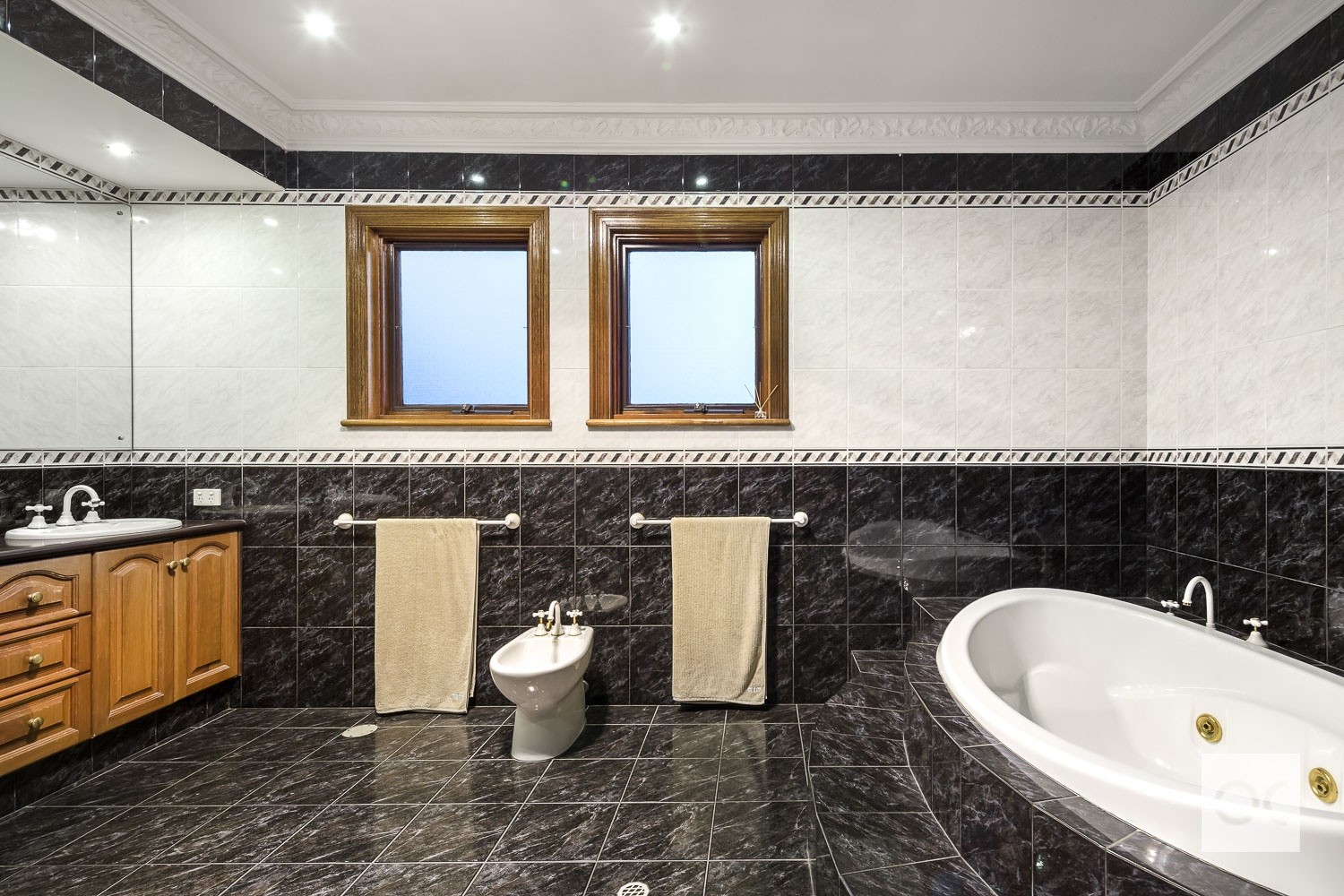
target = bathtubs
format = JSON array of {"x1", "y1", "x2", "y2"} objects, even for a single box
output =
[
  {"x1": 934, "y1": 586, "x2": 1344, "y2": 896},
  {"x1": 840, "y1": 518, "x2": 1035, "y2": 585}
]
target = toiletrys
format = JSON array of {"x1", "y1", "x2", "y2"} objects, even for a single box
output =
[{"x1": 1037, "y1": 564, "x2": 1053, "y2": 587}]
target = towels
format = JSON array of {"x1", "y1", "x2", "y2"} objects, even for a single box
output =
[
  {"x1": 373, "y1": 717, "x2": 477, "y2": 862},
  {"x1": 374, "y1": 517, "x2": 481, "y2": 714},
  {"x1": 671, "y1": 719, "x2": 772, "y2": 874},
  {"x1": 670, "y1": 516, "x2": 772, "y2": 707}
]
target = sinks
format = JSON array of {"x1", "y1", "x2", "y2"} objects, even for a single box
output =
[
  {"x1": 4, "y1": 518, "x2": 183, "y2": 540},
  {"x1": 488, "y1": 624, "x2": 595, "y2": 710},
  {"x1": 568, "y1": 594, "x2": 628, "y2": 626}
]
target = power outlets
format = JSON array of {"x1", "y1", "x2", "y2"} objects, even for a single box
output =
[{"x1": 192, "y1": 489, "x2": 222, "y2": 506}]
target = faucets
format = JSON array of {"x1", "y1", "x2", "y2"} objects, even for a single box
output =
[
  {"x1": 1012, "y1": 528, "x2": 1047, "y2": 559},
  {"x1": 545, "y1": 601, "x2": 565, "y2": 636},
  {"x1": 56, "y1": 484, "x2": 101, "y2": 527},
  {"x1": 576, "y1": 577, "x2": 593, "y2": 611},
  {"x1": 1181, "y1": 575, "x2": 1217, "y2": 631}
]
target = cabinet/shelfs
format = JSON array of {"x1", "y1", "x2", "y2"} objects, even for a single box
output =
[
  {"x1": 0, "y1": 554, "x2": 88, "y2": 777},
  {"x1": 90, "y1": 530, "x2": 243, "y2": 739}
]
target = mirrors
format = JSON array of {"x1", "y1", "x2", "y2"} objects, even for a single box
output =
[{"x1": 0, "y1": 153, "x2": 137, "y2": 466}]
